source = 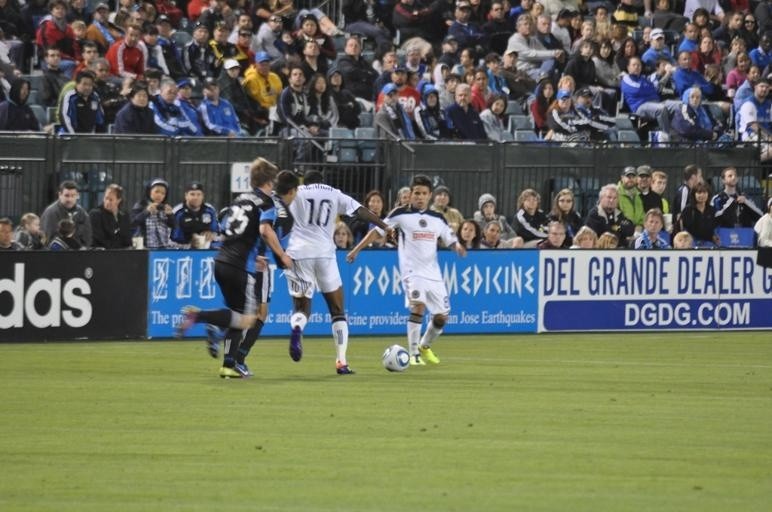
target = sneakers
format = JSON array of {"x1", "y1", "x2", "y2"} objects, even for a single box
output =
[
  {"x1": 218, "y1": 367, "x2": 244, "y2": 378},
  {"x1": 289, "y1": 325, "x2": 304, "y2": 362},
  {"x1": 207, "y1": 323, "x2": 228, "y2": 359},
  {"x1": 409, "y1": 353, "x2": 427, "y2": 366},
  {"x1": 175, "y1": 305, "x2": 202, "y2": 341},
  {"x1": 234, "y1": 363, "x2": 254, "y2": 376},
  {"x1": 337, "y1": 364, "x2": 356, "y2": 374},
  {"x1": 417, "y1": 344, "x2": 440, "y2": 364}
]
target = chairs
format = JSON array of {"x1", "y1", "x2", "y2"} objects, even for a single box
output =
[
  {"x1": 357, "y1": 112, "x2": 373, "y2": 128},
  {"x1": 614, "y1": 117, "x2": 635, "y2": 130},
  {"x1": 45, "y1": 107, "x2": 57, "y2": 128},
  {"x1": 354, "y1": 127, "x2": 377, "y2": 163},
  {"x1": 29, "y1": 104, "x2": 46, "y2": 127},
  {"x1": 25, "y1": 76, "x2": 40, "y2": 91},
  {"x1": 508, "y1": 114, "x2": 532, "y2": 131},
  {"x1": 25, "y1": 90, "x2": 38, "y2": 107},
  {"x1": 514, "y1": 130, "x2": 538, "y2": 143},
  {"x1": 328, "y1": 127, "x2": 358, "y2": 162},
  {"x1": 618, "y1": 129, "x2": 641, "y2": 147},
  {"x1": 504, "y1": 101, "x2": 525, "y2": 115}
]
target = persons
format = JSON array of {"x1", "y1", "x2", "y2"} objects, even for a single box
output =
[
  {"x1": 565, "y1": 40, "x2": 596, "y2": 86},
  {"x1": 574, "y1": 21, "x2": 597, "y2": 46},
  {"x1": 243, "y1": 51, "x2": 284, "y2": 107},
  {"x1": 644, "y1": 30, "x2": 673, "y2": 60},
  {"x1": 0, "y1": 77, "x2": 40, "y2": 129},
  {"x1": 652, "y1": 172, "x2": 669, "y2": 214},
  {"x1": 616, "y1": 167, "x2": 645, "y2": 248},
  {"x1": 738, "y1": 80, "x2": 772, "y2": 161},
  {"x1": 220, "y1": 59, "x2": 268, "y2": 133},
  {"x1": 107, "y1": 25, "x2": 147, "y2": 84},
  {"x1": 516, "y1": 190, "x2": 552, "y2": 241},
  {"x1": 669, "y1": 51, "x2": 718, "y2": 99},
  {"x1": 331, "y1": 35, "x2": 379, "y2": 102},
  {"x1": 415, "y1": 85, "x2": 451, "y2": 141},
  {"x1": 281, "y1": 171, "x2": 394, "y2": 375},
  {"x1": 709, "y1": 167, "x2": 764, "y2": 228},
  {"x1": 50, "y1": 219, "x2": 82, "y2": 250},
  {"x1": 680, "y1": 185, "x2": 716, "y2": 249},
  {"x1": 531, "y1": 78, "x2": 554, "y2": 133},
  {"x1": 443, "y1": 84, "x2": 485, "y2": 141},
  {"x1": 66, "y1": 19, "x2": 91, "y2": 60},
  {"x1": 74, "y1": 44, "x2": 101, "y2": 75},
  {"x1": 597, "y1": 232, "x2": 620, "y2": 249},
  {"x1": 548, "y1": 93, "x2": 590, "y2": 137},
  {"x1": 574, "y1": 85, "x2": 618, "y2": 143},
  {"x1": 670, "y1": 165, "x2": 703, "y2": 221},
  {"x1": 116, "y1": 87, "x2": 155, "y2": 132},
  {"x1": 670, "y1": 86, "x2": 718, "y2": 143},
  {"x1": 478, "y1": 90, "x2": 512, "y2": 143},
  {"x1": 480, "y1": 222, "x2": 523, "y2": 249},
  {"x1": 149, "y1": 80, "x2": 196, "y2": 134},
  {"x1": 178, "y1": 157, "x2": 293, "y2": 379},
  {"x1": 233, "y1": 25, "x2": 257, "y2": 59},
  {"x1": 679, "y1": 25, "x2": 730, "y2": 82},
  {"x1": 321, "y1": 70, "x2": 362, "y2": 131},
  {"x1": 508, "y1": 12, "x2": 565, "y2": 79},
  {"x1": 585, "y1": 184, "x2": 633, "y2": 249},
  {"x1": 631, "y1": 209, "x2": 672, "y2": 249},
  {"x1": 2, "y1": 1, "x2": 43, "y2": 81},
  {"x1": 621, "y1": 57, "x2": 684, "y2": 135},
  {"x1": 395, "y1": 186, "x2": 412, "y2": 209},
  {"x1": 169, "y1": 182, "x2": 219, "y2": 250},
  {"x1": 593, "y1": 37, "x2": 621, "y2": 88},
  {"x1": 1, "y1": 218, "x2": 24, "y2": 248},
  {"x1": 198, "y1": 77, "x2": 244, "y2": 136},
  {"x1": 536, "y1": 222, "x2": 571, "y2": 249},
  {"x1": 153, "y1": 13, "x2": 190, "y2": 80},
  {"x1": 62, "y1": 72, "x2": 104, "y2": 134},
  {"x1": 570, "y1": 225, "x2": 597, "y2": 248},
  {"x1": 86, "y1": 4, "x2": 125, "y2": 54},
  {"x1": 342, "y1": 0, "x2": 507, "y2": 74},
  {"x1": 674, "y1": 231, "x2": 695, "y2": 249},
  {"x1": 206, "y1": 169, "x2": 299, "y2": 379},
  {"x1": 546, "y1": 188, "x2": 582, "y2": 235},
  {"x1": 211, "y1": 18, "x2": 246, "y2": 67},
  {"x1": 297, "y1": 12, "x2": 330, "y2": 43},
  {"x1": 429, "y1": 186, "x2": 469, "y2": 247},
  {"x1": 93, "y1": 58, "x2": 124, "y2": 124},
  {"x1": 257, "y1": 15, "x2": 284, "y2": 57},
  {"x1": 36, "y1": 1, "x2": 75, "y2": 68},
  {"x1": 375, "y1": 66, "x2": 421, "y2": 121},
  {"x1": 373, "y1": 83, "x2": 416, "y2": 143},
  {"x1": 133, "y1": 179, "x2": 173, "y2": 249},
  {"x1": 39, "y1": 180, "x2": 92, "y2": 249},
  {"x1": 636, "y1": 165, "x2": 651, "y2": 196},
  {"x1": 753, "y1": 198, "x2": 771, "y2": 249},
  {"x1": 307, "y1": 74, "x2": 340, "y2": 130},
  {"x1": 355, "y1": 190, "x2": 393, "y2": 250},
  {"x1": 294, "y1": 41, "x2": 328, "y2": 73},
  {"x1": 90, "y1": 183, "x2": 133, "y2": 248},
  {"x1": 277, "y1": 67, "x2": 330, "y2": 162},
  {"x1": 455, "y1": 218, "x2": 486, "y2": 250},
  {"x1": 181, "y1": 18, "x2": 219, "y2": 75},
  {"x1": 508, "y1": 0, "x2": 770, "y2": 25},
  {"x1": 34, "y1": 47, "x2": 67, "y2": 106},
  {"x1": 139, "y1": 26, "x2": 168, "y2": 77},
  {"x1": 334, "y1": 222, "x2": 354, "y2": 251},
  {"x1": 556, "y1": 75, "x2": 576, "y2": 102},
  {"x1": 115, "y1": 2, "x2": 322, "y2": 29},
  {"x1": 16, "y1": 214, "x2": 46, "y2": 250},
  {"x1": 722, "y1": 27, "x2": 771, "y2": 100},
  {"x1": 346, "y1": 174, "x2": 468, "y2": 366},
  {"x1": 622, "y1": 38, "x2": 643, "y2": 60},
  {"x1": 280, "y1": 32, "x2": 299, "y2": 58},
  {"x1": 473, "y1": 193, "x2": 516, "y2": 240}
]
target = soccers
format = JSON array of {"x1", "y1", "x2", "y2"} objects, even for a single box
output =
[{"x1": 381, "y1": 344, "x2": 411, "y2": 373}]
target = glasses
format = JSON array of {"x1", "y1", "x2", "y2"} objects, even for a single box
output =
[
  {"x1": 493, "y1": 7, "x2": 504, "y2": 11},
  {"x1": 239, "y1": 32, "x2": 253, "y2": 38},
  {"x1": 558, "y1": 199, "x2": 573, "y2": 203},
  {"x1": 744, "y1": 20, "x2": 756, "y2": 24}
]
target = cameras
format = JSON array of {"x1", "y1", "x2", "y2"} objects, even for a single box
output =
[{"x1": 157, "y1": 203, "x2": 166, "y2": 209}]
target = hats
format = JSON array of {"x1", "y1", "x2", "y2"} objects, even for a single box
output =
[
  {"x1": 477, "y1": 193, "x2": 497, "y2": 210},
  {"x1": 620, "y1": 166, "x2": 637, "y2": 177},
  {"x1": 442, "y1": 33, "x2": 458, "y2": 43},
  {"x1": 382, "y1": 82, "x2": 398, "y2": 95},
  {"x1": 484, "y1": 52, "x2": 502, "y2": 62},
  {"x1": 556, "y1": 90, "x2": 570, "y2": 99},
  {"x1": 391, "y1": 64, "x2": 409, "y2": 73},
  {"x1": 187, "y1": 183, "x2": 204, "y2": 190},
  {"x1": 223, "y1": 58, "x2": 242, "y2": 70},
  {"x1": 503, "y1": 48, "x2": 520, "y2": 58},
  {"x1": 176, "y1": 78, "x2": 194, "y2": 88},
  {"x1": 155, "y1": 14, "x2": 170, "y2": 24},
  {"x1": 636, "y1": 165, "x2": 651, "y2": 176},
  {"x1": 254, "y1": 51, "x2": 271, "y2": 64},
  {"x1": 648, "y1": 27, "x2": 666, "y2": 40},
  {"x1": 201, "y1": 76, "x2": 217, "y2": 88},
  {"x1": 456, "y1": 0, "x2": 472, "y2": 8},
  {"x1": 575, "y1": 87, "x2": 594, "y2": 98},
  {"x1": 94, "y1": 1, "x2": 110, "y2": 11}
]
